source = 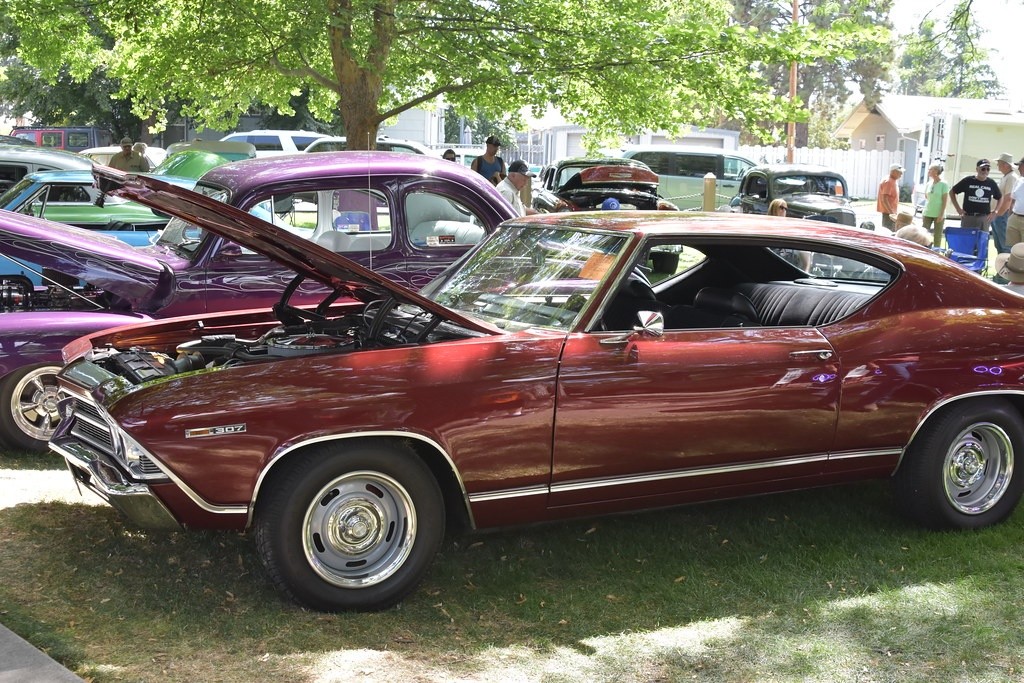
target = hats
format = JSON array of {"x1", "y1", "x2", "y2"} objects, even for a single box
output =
[
  {"x1": 995, "y1": 242, "x2": 1024, "y2": 283},
  {"x1": 486, "y1": 135, "x2": 501, "y2": 146},
  {"x1": 508, "y1": 159, "x2": 536, "y2": 178},
  {"x1": 1014, "y1": 158, "x2": 1024, "y2": 165},
  {"x1": 121, "y1": 138, "x2": 133, "y2": 147},
  {"x1": 602, "y1": 197, "x2": 620, "y2": 210},
  {"x1": 977, "y1": 159, "x2": 991, "y2": 167},
  {"x1": 891, "y1": 164, "x2": 906, "y2": 172},
  {"x1": 994, "y1": 153, "x2": 1017, "y2": 169}
]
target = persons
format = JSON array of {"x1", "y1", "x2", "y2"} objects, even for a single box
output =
[
  {"x1": 773, "y1": 249, "x2": 810, "y2": 272},
  {"x1": 767, "y1": 199, "x2": 787, "y2": 216},
  {"x1": 497, "y1": 159, "x2": 536, "y2": 216},
  {"x1": 471, "y1": 136, "x2": 506, "y2": 186},
  {"x1": 877, "y1": 164, "x2": 905, "y2": 229},
  {"x1": 895, "y1": 211, "x2": 931, "y2": 246},
  {"x1": 657, "y1": 201, "x2": 679, "y2": 211},
  {"x1": 990, "y1": 154, "x2": 1019, "y2": 253},
  {"x1": 860, "y1": 221, "x2": 875, "y2": 231},
  {"x1": 923, "y1": 165, "x2": 948, "y2": 248},
  {"x1": 443, "y1": 149, "x2": 455, "y2": 162},
  {"x1": 44, "y1": 136, "x2": 52, "y2": 147},
  {"x1": 1005, "y1": 156, "x2": 1024, "y2": 246},
  {"x1": 995, "y1": 242, "x2": 1024, "y2": 284},
  {"x1": 949, "y1": 159, "x2": 1003, "y2": 233},
  {"x1": 109, "y1": 138, "x2": 150, "y2": 171}
]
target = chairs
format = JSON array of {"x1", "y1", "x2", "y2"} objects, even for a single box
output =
[
  {"x1": 608, "y1": 278, "x2": 668, "y2": 330},
  {"x1": 347, "y1": 235, "x2": 384, "y2": 251},
  {"x1": 317, "y1": 230, "x2": 345, "y2": 251},
  {"x1": 932, "y1": 226, "x2": 992, "y2": 277},
  {"x1": 662, "y1": 287, "x2": 763, "y2": 328}
]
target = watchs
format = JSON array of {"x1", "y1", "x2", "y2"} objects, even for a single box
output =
[{"x1": 994, "y1": 211, "x2": 998, "y2": 213}]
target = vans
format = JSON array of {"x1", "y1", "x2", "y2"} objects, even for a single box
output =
[{"x1": 622, "y1": 146, "x2": 759, "y2": 213}]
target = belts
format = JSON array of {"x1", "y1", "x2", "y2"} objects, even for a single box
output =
[
  {"x1": 965, "y1": 213, "x2": 988, "y2": 216},
  {"x1": 1014, "y1": 213, "x2": 1024, "y2": 217}
]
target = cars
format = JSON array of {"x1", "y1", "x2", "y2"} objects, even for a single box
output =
[
  {"x1": 0, "y1": 151, "x2": 651, "y2": 457},
  {"x1": 0, "y1": 126, "x2": 571, "y2": 287},
  {"x1": 731, "y1": 163, "x2": 875, "y2": 231},
  {"x1": 530, "y1": 155, "x2": 680, "y2": 216},
  {"x1": 45, "y1": 167, "x2": 1024, "y2": 613}
]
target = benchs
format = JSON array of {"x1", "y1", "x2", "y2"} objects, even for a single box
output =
[
  {"x1": 693, "y1": 271, "x2": 874, "y2": 324},
  {"x1": 410, "y1": 220, "x2": 483, "y2": 245}
]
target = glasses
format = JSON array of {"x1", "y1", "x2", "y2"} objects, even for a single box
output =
[
  {"x1": 446, "y1": 158, "x2": 456, "y2": 162},
  {"x1": 779, "y1": 205, "x2": 788, "y2": 213},
  {"x1": 978, "y1": 167, "x2": 990, "y2": 172}
]
